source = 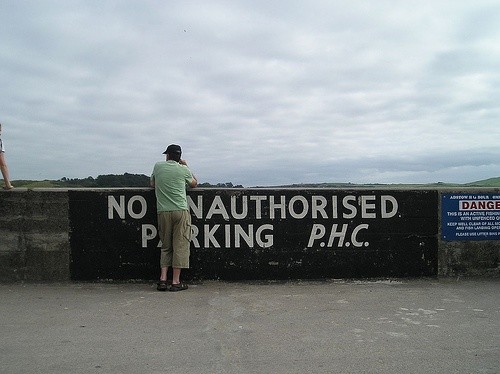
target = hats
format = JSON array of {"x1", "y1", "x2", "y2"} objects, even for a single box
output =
[{"x1": 162, "y1": 145, "x2": 182, "y2": 155}]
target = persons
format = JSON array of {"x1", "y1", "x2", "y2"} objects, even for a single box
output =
[
  {"x1": 150, "y1": 144, "x2": 198, "y2": 292},
  {"x1": 0, "y1": 124, "x2": 13, "y2": 190}
]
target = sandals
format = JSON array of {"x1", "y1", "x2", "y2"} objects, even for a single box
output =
[
  {"x1": 169, "y1": 282, "x2": 188, "y2": 291},
  {"x1": 157, "y1": 281, "x2": 167, "y2": 291}
]
